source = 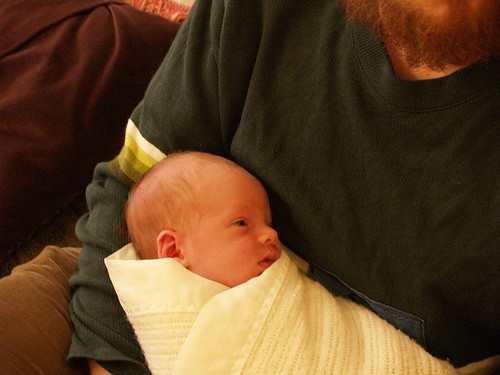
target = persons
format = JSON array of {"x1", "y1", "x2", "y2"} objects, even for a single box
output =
[
  {"x1": 105, "y1": 151, "x2": 457, "y2": 374},
  {"x1": 0, "y1": 1, "x2": 500, "y2": 374}
]
